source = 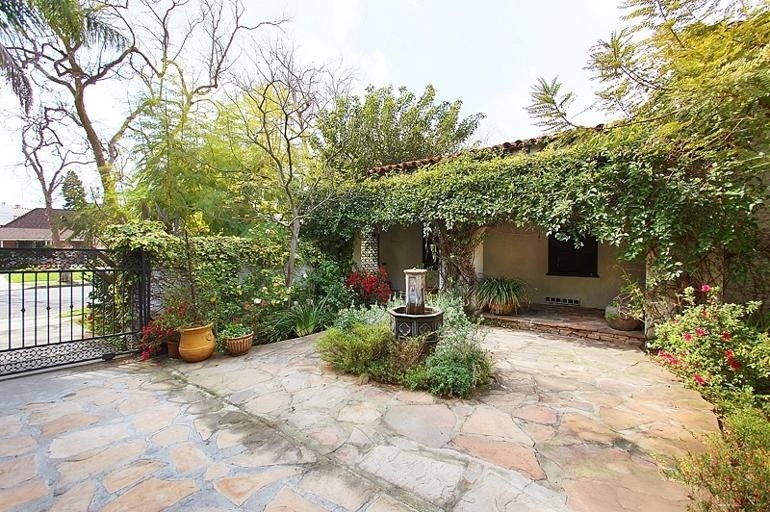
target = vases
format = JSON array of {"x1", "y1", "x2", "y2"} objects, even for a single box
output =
[{"x1": 177, "y1": 322, "x2": 216, "y2": 363}]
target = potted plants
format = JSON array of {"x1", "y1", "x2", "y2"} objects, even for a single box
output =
[
  {"x1": 215, "y1": 319, "x2": 254, "y2": 356},
  {"x1": 464, "y1": 273, "x2": 538, "y2": 317}
]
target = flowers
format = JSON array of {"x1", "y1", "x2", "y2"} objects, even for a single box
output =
[{"x1": 137, "y1": 299, "x2": 206, "y2": 361}]
